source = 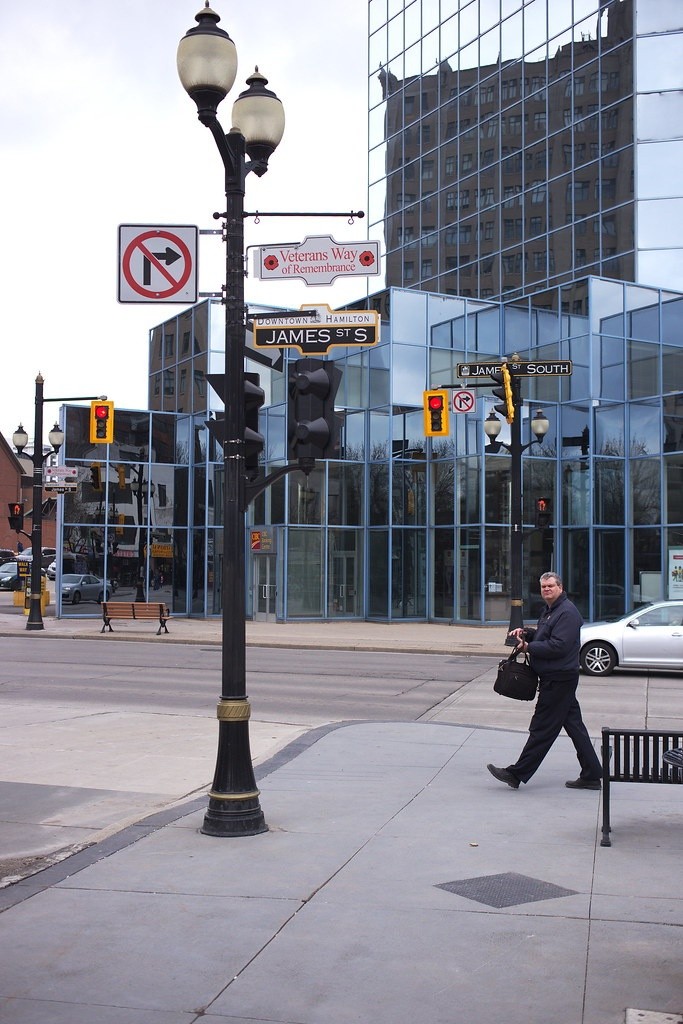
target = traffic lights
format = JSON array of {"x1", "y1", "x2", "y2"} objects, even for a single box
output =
[
  {"x1": 89, "y1": 461, "x2": 103, "y2": 491},
  {"x1": 489, "y1": 369, "x2": 516, "y2": 426},
  {"x1": 422, "y1": 390, "x2": 450, "y2": 437},
  {"x1": 90, "y1": 401, "x2": 114, "y2": 443},
  {"x1": 534, "y1": 495, "x2": 552, "y2": 530},
  {"x1": 6, "y1": 503, "x2": 28, "y2": 531}
]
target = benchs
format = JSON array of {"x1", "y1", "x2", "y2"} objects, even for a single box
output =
[
  {"x1": 100, "y1": 601, "x2": 172, "y2": 636},
  {"x1": 601, "y1": 726, "x2": 682, "y2": 846}
]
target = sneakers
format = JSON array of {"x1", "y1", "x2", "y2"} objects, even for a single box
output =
[
  {"x1": 565, "y1": 777, "x2": 601, "y2": 790},
  {"x1": 488, "y1": 764, "x2": 520, "y2": 788}
]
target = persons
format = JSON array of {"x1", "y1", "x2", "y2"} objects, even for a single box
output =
[
  {"x1": 612, "y1": 567, "x2": 624, "y2": 585},
  {"x1": 672, "y1": 565, "x2": 683, "y2": 582},
  {"x1": 668, "y1": 612, "x2": 681, "y2": 625},
  {"x1": 445, "y1": 567, "x2": 453, "y2": 593},
  {"x1": 17, "y1": 541, "x2": 24, "y2": 554},
  {"x1": 487, "y1": 571, "x2": 603, "y2": 790},
  {"x1": 460, "y1": 570, "x2": 465, "y2": 590},
  {"x1": 135, "y1": 566, "x2": 159, "y2": 602}
]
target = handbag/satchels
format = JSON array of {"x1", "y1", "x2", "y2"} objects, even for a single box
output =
[{"x1": 494, "y1": 634, "x2": 538, "y2": 700}]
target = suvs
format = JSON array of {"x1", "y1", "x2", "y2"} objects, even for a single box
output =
[{"x1": 16, "y1": 547, "x2": 56, "y2": 568}]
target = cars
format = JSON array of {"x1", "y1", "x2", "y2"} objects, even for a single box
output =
[
  {"x1": 0, "y1": 549, "x2": 17, "y2": 566},
  {"x1": 62, "y1": 574, "x2": 119, "y2": 604},
  {"x1": 593, "y1": 584, "x2": 652, "y2": 617},
  {"x1": 579, "y1": 599, "x2": 683, "y2": 676},
  {"x1": 0, "y1": 562, "x2": 46, "y2": 591},
  {"x1": 47, "y1": 553, "x2": 90, "y2": 580}
]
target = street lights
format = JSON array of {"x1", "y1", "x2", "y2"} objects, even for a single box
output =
[
  {"x1": 562, "y1": 424, "x2": 602, "y2": 586},
  {"x1": 13, "y1": 373, "x2": 65, "y2": 630},
  {"x1": 130, "y1": 446, "x2": 155, "y2": 602},
  {"x1": 483, "y1": 353, "x2": 549, "y2": 648},
  {"x1": 176, "y1": 0, "x2": 286, "y2": 838}
]
